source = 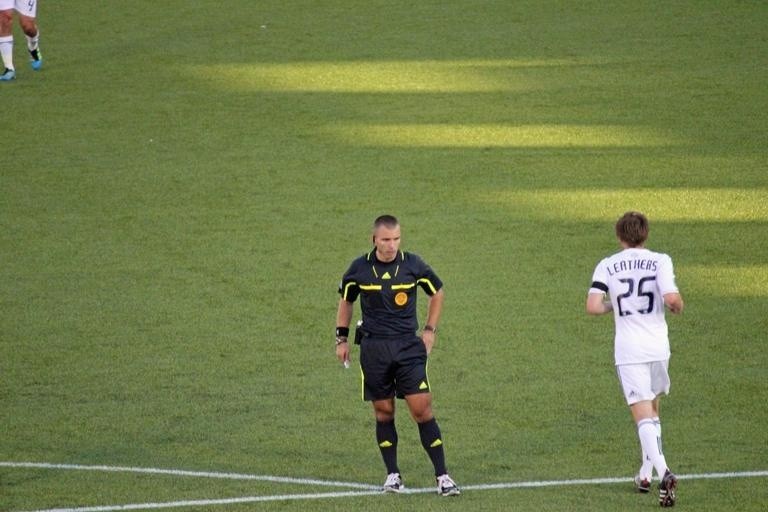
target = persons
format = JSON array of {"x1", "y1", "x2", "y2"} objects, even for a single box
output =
[
  {"x1": 0, "y1": 0, "x2": 43, "y2": 81},
  {"x1": 586, "y1": 212, "x2": 685, "y2": 507},
  {"x1": 335, "y1": 215, "x2": 461, "y2": 497}
]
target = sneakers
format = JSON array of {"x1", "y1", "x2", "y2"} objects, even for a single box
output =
[
  {"x1": 659, "y1": 470, "x2": 677, "y2": 507},
  {"x1": 0, "y1": 68, "x2": 17, "y2": 81},
  {"x1": 635, "y1": 473, "x2": 651, "y2": 492},
  {"x1": 28, "y1": 46, "x2": 42, "y2": 71},
  {"x1": 437, "y1": 474, "x2": 461, "y2": 496},
  {"x1": 384, "y1": 472, "x2": 405, "y2": 490}
]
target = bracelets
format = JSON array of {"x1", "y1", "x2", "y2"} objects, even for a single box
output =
[
  {"x1": 423, "y1": 325, "x2": 436, "y2": 334},
  {"x1": 336, "y1": 337, "x2": 347, "y2": 345},
  {"x1": 336, "y1": 326, "x2": 349, "y2": 337}
]
